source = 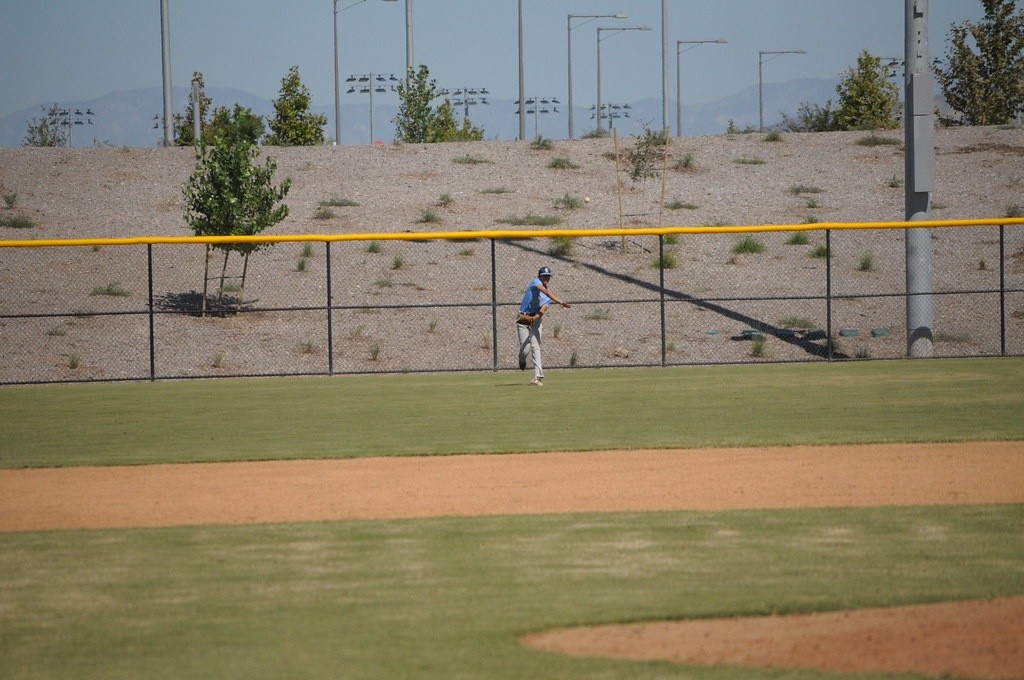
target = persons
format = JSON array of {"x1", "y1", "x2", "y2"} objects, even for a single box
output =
[{"x1": 516, "y1": 267, "x2": 571, "y2": 386}]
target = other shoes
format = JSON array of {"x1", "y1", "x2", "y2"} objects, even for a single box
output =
[
  {"x1": 519, "y1": 361, "x2": 526, "y2": 370},
  {"x1": 531, "y1": 379, "x2": 543, "y2": 386}
]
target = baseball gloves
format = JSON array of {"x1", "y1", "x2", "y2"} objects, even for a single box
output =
[{"x1": 516, "y1": 313, "x2": 535, "y2": 327}]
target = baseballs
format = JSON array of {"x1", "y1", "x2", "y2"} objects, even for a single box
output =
[{"x1": 584, "y1": 197, "x2": 590, "y2": 204}]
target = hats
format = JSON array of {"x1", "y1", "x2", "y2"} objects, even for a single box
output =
[{"x1": 539, "y1": 267, "x2": 552, "y2": 276}]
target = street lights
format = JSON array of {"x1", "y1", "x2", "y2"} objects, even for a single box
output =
[
  {"x1": 758, "y1": 49, "x2": 807, "y2": 132},
  {"x1": 514, "y1": 97, "x2": 560, "y2": 140},
  {"x1": 590, "y1": 103, "x2": 632, "y2": 137},
  {"x1": 346, "y1": 73, "x2": 399, "y2": 144},
  {"x1": 44, "y1": 103, "x2": 97, "y2": 147},
  {"x1": 676, "y1": 38, "x2": 730, "y2": 137},
  {"x1": 596, "y1": 24, "x2": 653, "y2": 134},
  {"x1": 566, "y1": 13, "x2": 628, "y2": 135},
  {"x1": 438, "y1": 86, "x2": 489, "y2": 140}
]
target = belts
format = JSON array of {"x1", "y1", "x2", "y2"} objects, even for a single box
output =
[{"x1": 521, "y1": 313, "x2": 535, "y2": 316}]
